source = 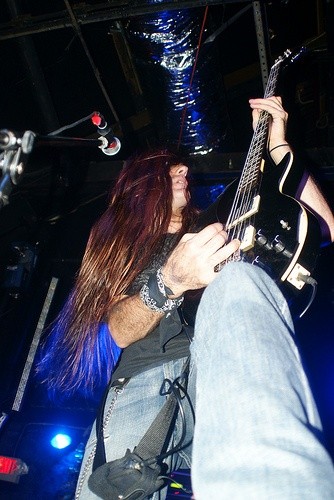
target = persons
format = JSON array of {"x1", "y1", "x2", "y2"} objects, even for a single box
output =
[{"x1": 33, "y1": 96, "x2": 334, "y2": 500}]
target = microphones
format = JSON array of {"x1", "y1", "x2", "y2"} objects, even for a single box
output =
[{"x1": 91, "y1": 111, "x2": 122, "y2": 156}]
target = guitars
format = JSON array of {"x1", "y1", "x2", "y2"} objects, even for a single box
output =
[{"x1": 181, "y1": 45, "x2": 322, "y2": 320}]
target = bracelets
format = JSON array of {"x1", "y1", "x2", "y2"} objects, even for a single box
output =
[
  {"x1": 139, "y1": 269, "x2": 186, "y2": 318},
  {"x1": 268, "y1": 143, "x2": 289, "y2": 154}
]
